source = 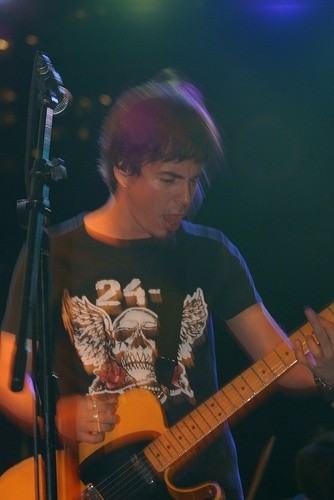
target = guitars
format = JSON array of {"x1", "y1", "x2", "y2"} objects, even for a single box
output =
[{"x1": 1, "y1": 298, "x2": 334, "y2": 500}]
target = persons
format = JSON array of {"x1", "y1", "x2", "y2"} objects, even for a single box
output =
[{"x1": 1, "y1": 81, "x2": 334, "y2": 499}]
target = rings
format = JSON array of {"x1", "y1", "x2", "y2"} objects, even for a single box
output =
[{"x1": 92, "y1": 403, "x2": 98, "y2": 418}]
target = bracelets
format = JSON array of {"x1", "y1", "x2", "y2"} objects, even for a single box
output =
[{"x1": 314, "y1": 375, "x2": 334, "y2": 392}]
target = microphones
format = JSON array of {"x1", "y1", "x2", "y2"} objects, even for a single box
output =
[{"x1": 36, "y1": 52, "x2": 74, "y2": 115}]
